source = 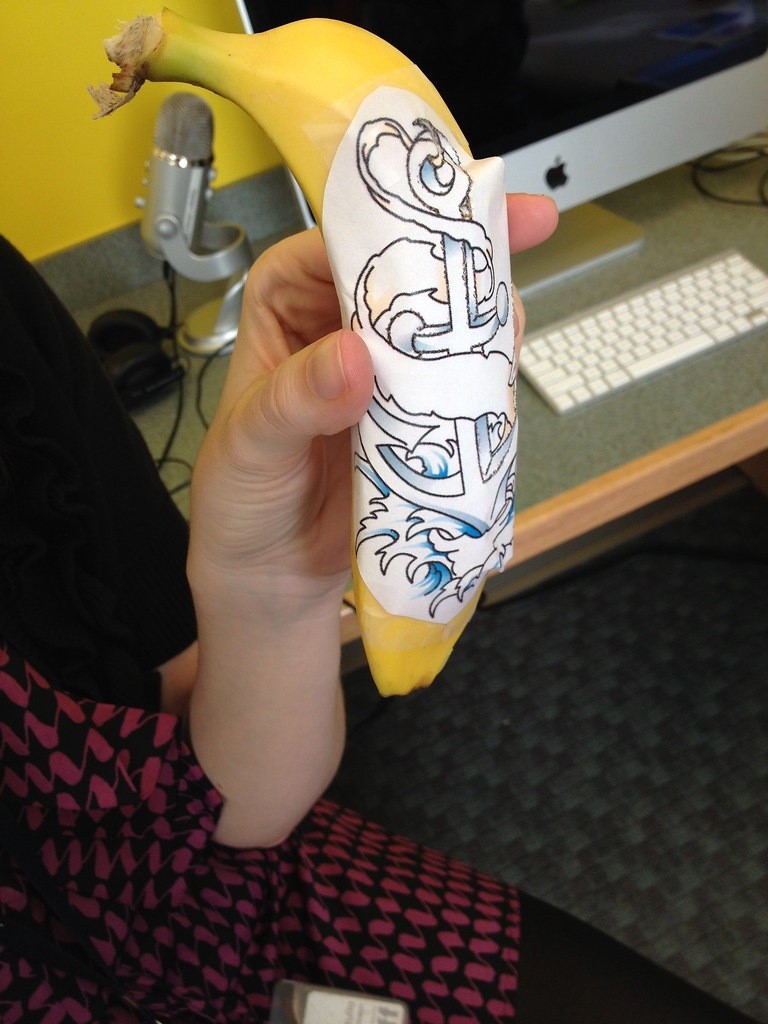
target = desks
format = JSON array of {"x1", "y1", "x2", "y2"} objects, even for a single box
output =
[{"x1": 69, "y1": 153, "x2": 768, "y2": 618}]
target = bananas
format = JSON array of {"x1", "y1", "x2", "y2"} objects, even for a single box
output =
[{"x1": 88, "y1": 6, "x2": 512, "y2": 698}]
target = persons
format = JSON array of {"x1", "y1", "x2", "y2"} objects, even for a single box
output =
[{"x1": 0, "y1": 193, "x2": 767, "y2": 1024}]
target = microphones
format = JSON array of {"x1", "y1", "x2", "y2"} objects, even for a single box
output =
[{"x1": 134, "y1": 92, "x2": 216, "y2": 260}]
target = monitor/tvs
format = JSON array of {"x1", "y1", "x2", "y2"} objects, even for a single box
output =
[{"x1": 236, "y1": 0, "x2": 768, "y2": 300}]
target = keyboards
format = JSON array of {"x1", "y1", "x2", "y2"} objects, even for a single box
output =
[{"x1": 512, "y1": 249, "x2": 768, "y2": 415}]
